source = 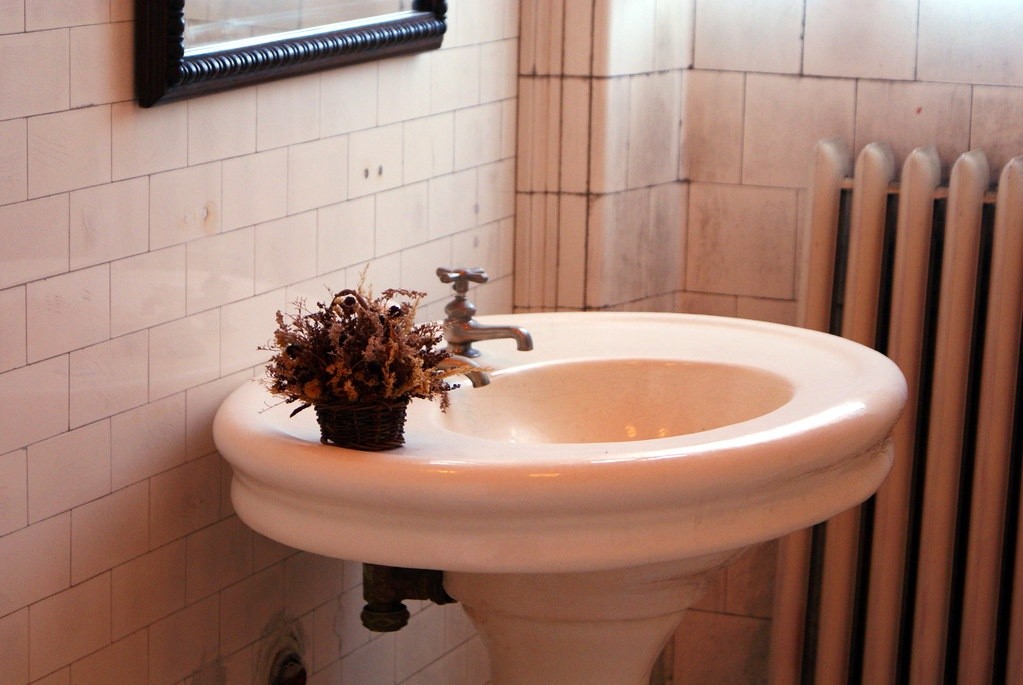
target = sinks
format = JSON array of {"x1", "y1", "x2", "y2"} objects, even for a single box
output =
[{"x1": 210, "y1": 303, "x2": 913, "y2": 590}]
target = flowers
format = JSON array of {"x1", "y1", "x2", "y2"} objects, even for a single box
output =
[{"x1": 253, "y1": 255, "x2": 495, "y2": 413}]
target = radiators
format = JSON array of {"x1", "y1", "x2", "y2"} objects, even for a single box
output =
[{"x1": 765, "y1": 135, "x2": 1022, "y2": 681}]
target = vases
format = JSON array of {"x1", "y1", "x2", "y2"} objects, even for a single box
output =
[{"x1": 312, "y1": 397, "x2": 407, "y2": 451}]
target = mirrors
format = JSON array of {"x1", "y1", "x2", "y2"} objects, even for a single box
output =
[{"x1": 132, "y1": 0, "x2": 447, "y2": 108}]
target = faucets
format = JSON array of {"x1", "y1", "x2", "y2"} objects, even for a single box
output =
[{"x1": 431, "y1": 264, "x2": 537, "y2": 359}]
